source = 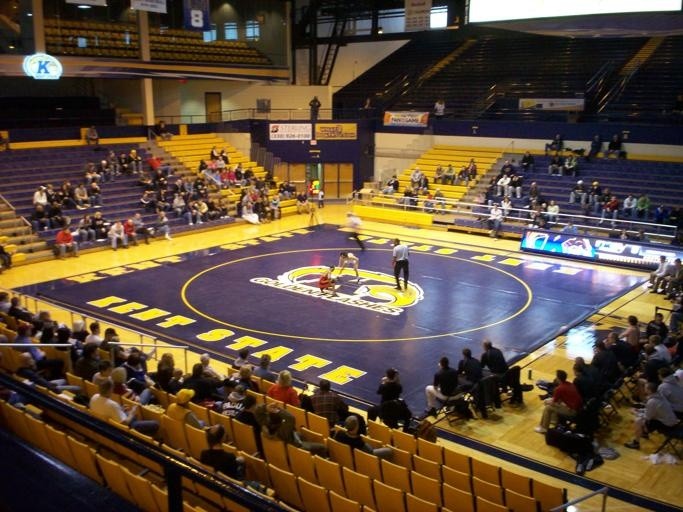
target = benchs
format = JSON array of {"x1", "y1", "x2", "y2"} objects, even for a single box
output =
[
  {"x1": 159, "y1": 134, "x2": 309, "y2": 235},
  {"x1": 0, "y1": 95, "x2": 159, "y2": 271}
]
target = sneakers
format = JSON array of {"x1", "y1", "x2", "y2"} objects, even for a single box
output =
[
  {"x1": 647, "y1": 281, "x2": 675, "y2": 300},
  {"x1": 640, "y1": 432, "x2": 649, "y2": 439},
  {"x1": 534, "y1": 426, "x2": 547, "y2": 433},
  {"x1": 623, "y1": 437, "x2": 640, "y2": 450},
  {"x1": 423, "y1": 406, "x2": 437, "y2": 416}
]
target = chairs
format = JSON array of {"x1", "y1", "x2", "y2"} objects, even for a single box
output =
[
  {"x1": 437, "y1": 366, "x2": 526, "y2": 428},
  {"x1": 572, "y1": 341, "x2": 682, "y2": 456},
  {"x1": 109, "y1": 367, "x2": 301, "y2": 412},
  {"x1": 40, "y1": 17, "x2": 274, "y2": 67},
  {"x1": 0, "y1": 312, "x2": 109, "y2": 412}
]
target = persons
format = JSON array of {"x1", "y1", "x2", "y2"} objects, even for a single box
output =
[
  {"x1": 335, "y1": 250, "x2": 363, "y2": 286},
  {"x1": 475, "y1": 338, "x2": 506, "y2": 383},
  {"x1": 30, "y1": 146, "x2": 326, "y2": 258},
  {"x1": 381, "y1": 159, "x2": 477, "y2": 215},
  {"x1": 0, "y1": 293, "x2": 412, "y2": 484},
  {"x1": 340, "y1": 211, "x2": 366, "y2": 253},
  {"x1": 306, "y1": 95, "x2": 320, "y2": 122},
  {"x1": 433, "y1": 96, "x2": 446, "y2": 122},
  {"x1": 454, "y1": 349, "x2": 482, "y2": 391},
  {"x1": 534, "y1": 254, "x2": 682, "y2": 482},
  {"x1": 0, "y1": 136, "x2": 9, "y2": 152},
  {"x1": 391, "y1": 237, "x2": 410, "y2": 292},
  {"x1": 475, "y1": 133, "x2": 682, "y2": 247},
  {"x1": 317, "y1": 265, "x2": 339, "y2": 298},
  {"x1": 423, "y1": 356, "x2": 457, "y2": 413},
  {"x1": 85, "y1": 125, "x2": 100, "y2": 146},
  {"x1": 0, "y1": 243, "x2": 14, "y2": 267},
  {"x1": 158, "y1": 121, "x2": 173, "y2": 142}
]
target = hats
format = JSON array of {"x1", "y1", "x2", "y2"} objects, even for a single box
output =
[{"x1": 174, "y1": 388, "x2": 196, "y2": 405}]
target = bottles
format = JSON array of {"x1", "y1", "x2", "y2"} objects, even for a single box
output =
[{"x1": 528, "y1": 369, "x2": 531, "y2": 379}]
